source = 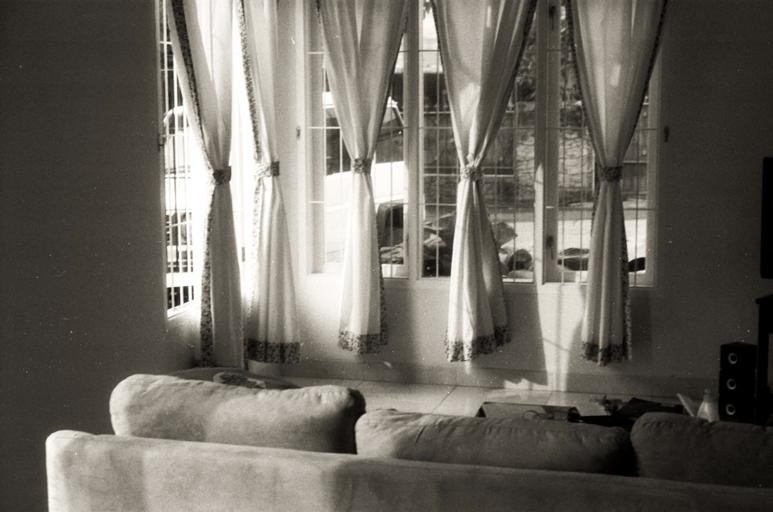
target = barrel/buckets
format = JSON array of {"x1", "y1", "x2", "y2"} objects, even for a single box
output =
[{"x1": 695, "y1": 388, "x2": 720, "y2": 423}]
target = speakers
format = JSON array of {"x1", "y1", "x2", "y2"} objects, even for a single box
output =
[
  {"x1": 720, "y1": 342, "x2": 756, "y2": 374},
  {"x1": 719, "y1": 397, "x2": 754, "y2": 422},
  {"x1": 719, "y1": 372, "x2": 755, "y2": 397}
]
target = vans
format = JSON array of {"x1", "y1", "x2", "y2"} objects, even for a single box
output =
[{"x1": 162, "y1": 91, "x2": 401, "y2": 290}]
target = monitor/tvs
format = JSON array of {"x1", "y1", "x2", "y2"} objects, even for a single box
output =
[{"x1": 760, "y1": 157, "x2": 773, "y2": 279}]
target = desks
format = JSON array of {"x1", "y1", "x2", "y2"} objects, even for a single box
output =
[{"x1": 752, "y1": 295, "x2": 773, "y2": 426}]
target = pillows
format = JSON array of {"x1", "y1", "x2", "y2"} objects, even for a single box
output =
[
  {"x1": 635, "y1": 413, "x2": 770, "y2": 488},
  {"x1": 109, "y1": 374, "x2": 364, "y2": 456},
  {"x1": 355, "y1": 403, "x2": 632, "y2": 476}
]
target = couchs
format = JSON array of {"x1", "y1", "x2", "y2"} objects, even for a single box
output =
[{"x1": 46, "y1": 427, "x2": 772, "y2": 512}]
